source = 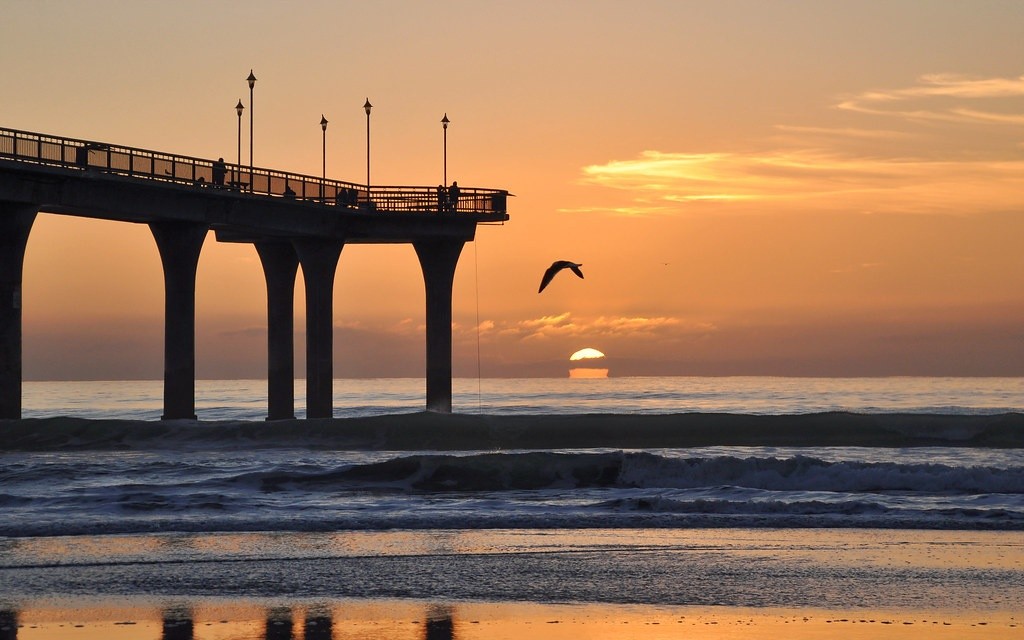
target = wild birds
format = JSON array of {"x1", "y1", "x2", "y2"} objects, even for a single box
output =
[{"x1": 537, "y1": 260, "x2": 585, "y2": 294}]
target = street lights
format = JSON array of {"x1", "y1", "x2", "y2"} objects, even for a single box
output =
[
  {"x1": 235, "y1": 99, "x2": 245, "y2": 182},
  {"x1": 319, "y1": 114, "x2": 328, "y2": 200},
  {"x1": 245, "y1": 68, "x2": 259, "y2": 192},
  {"x1": 441, "y1": 113, "x2": 451, "y2": 212},
  {"x1": 362, "y1": 98, "x2": 375, "y2": 204}
]
